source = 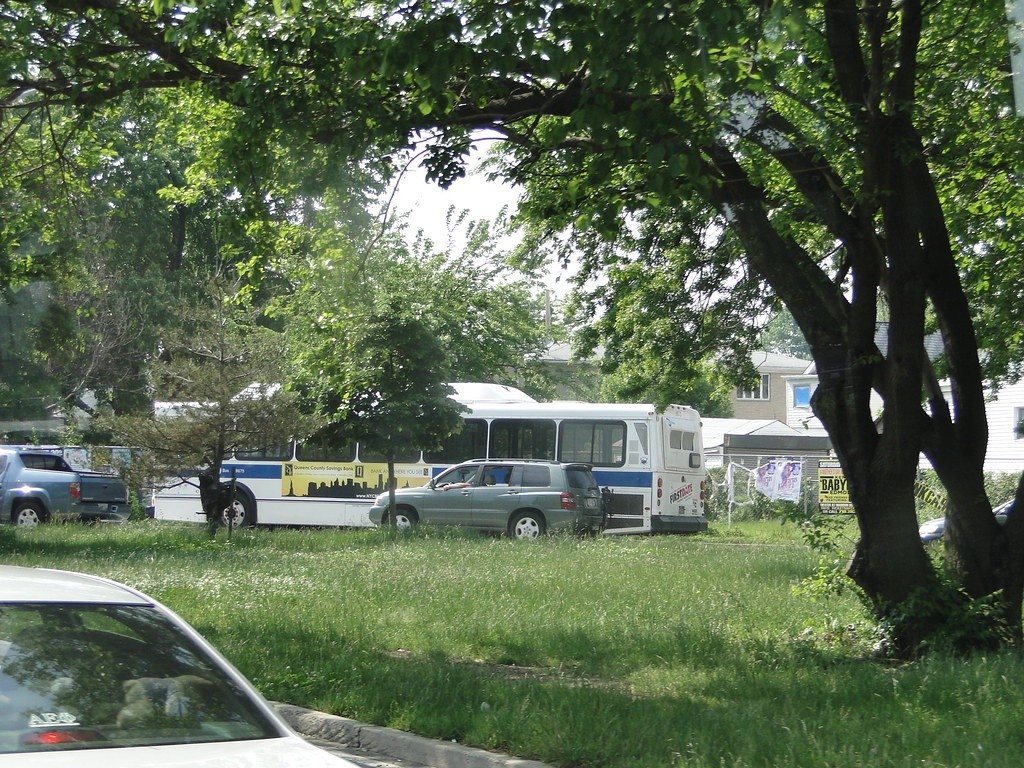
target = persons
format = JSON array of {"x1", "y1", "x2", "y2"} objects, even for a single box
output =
[{"x1": 444, "y1": 474, "x2": 487, "y2": 491}]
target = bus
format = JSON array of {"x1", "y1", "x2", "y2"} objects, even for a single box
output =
[{"x1": 154, "y1": 382, "x2": 709, "y2": 538}]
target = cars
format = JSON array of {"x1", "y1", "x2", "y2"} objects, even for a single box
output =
[
  {"x1": 919, "y1": 499, "x2": 1016, "y2": 545},
  {"x1": 1, "y1": 565, "x2": 362, "y2": 768}
]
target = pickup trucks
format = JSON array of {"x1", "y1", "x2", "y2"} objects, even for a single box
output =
[{"x1": 0, "y1": 449, "x2": 126, "y2": 526}]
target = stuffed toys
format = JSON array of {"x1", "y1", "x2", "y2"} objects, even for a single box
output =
[{"x1": 40, "y1": 673, "x2": 213, "y2": 730}]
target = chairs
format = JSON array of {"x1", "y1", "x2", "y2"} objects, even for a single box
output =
[{"x1": 486, "y1": 474, "x2": 496, "y2": 484}]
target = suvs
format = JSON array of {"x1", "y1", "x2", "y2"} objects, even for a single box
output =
[{"x1": 368, "y1": 459, "x2": 608, "y2": 542}]
